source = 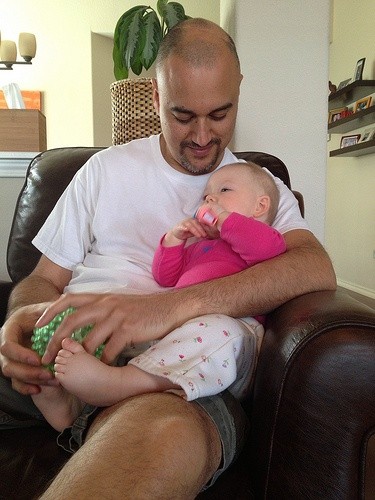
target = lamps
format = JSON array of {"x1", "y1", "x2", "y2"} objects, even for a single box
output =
[{"x1": 0, "y1": 31, "x2": 36, "y2": 70}]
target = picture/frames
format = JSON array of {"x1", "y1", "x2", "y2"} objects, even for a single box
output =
[
  {"x1": 354, "y1": 58, "x2": 365, "y2": 80},
  {"x1": 340, "y1": 134, "x2": 361, "y2": 148},
  {"x1": 353, "y1": 97, "x2": 372, "y2": 113},
  {"x1": 357, "y1": 128, "x2": 375, "y2": 144},
  {"x1": 329, "y1": 107, "x2": 348, "y2": 124}
]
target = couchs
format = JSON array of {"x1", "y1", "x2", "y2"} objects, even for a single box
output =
[{"x1": 0, "y1": 147, "x2": 375, "y2": 499}]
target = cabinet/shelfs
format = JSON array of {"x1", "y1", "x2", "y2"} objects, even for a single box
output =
[{"x1": 328, "y1": 80, "x2": 375, "y2": 158}]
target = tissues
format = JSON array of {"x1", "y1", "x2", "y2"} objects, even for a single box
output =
[{"x1": 0, "y1": 82, "x2": 47, "y2": 153}]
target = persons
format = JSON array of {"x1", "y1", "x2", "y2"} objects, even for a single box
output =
[
  {"x1": 29, "y1": 162, "x2": 287, "y2": 433},
  {"x1": 1, "y1": 18, "x2": 338, "y2": 496}
]
